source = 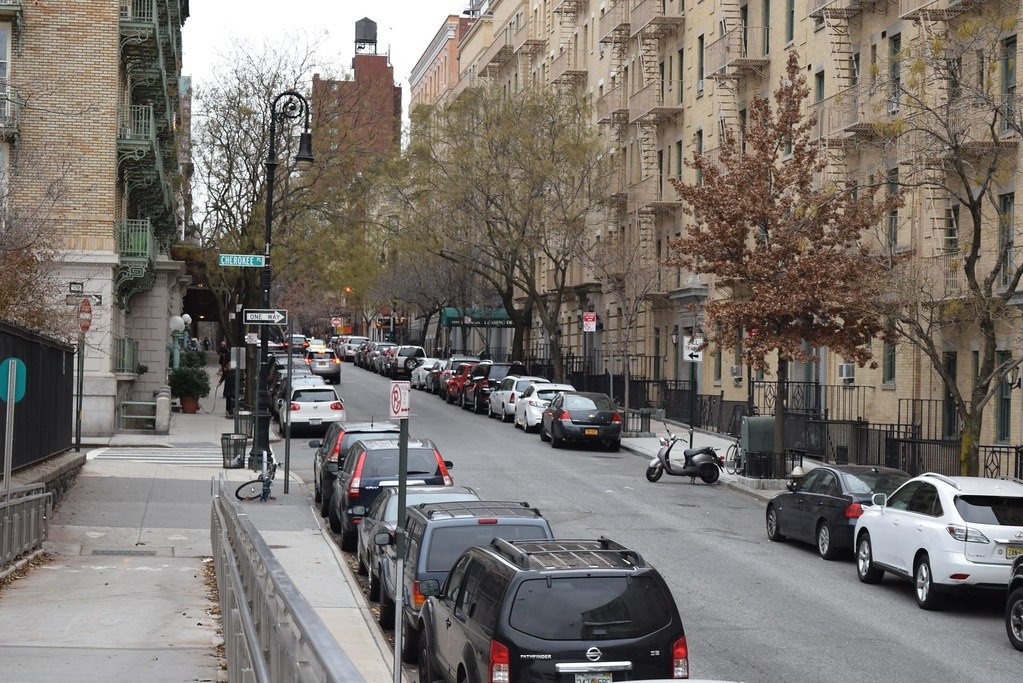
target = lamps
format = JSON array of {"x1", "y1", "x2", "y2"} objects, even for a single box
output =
[
  {"x1": 577, "y1": 319, "x2": 582, "y2": 332},
  {"x1": 670, "y1": 331, "x2": 679, "y2": 348},
  {"x1": 1004, "y1": 359, "x2": 1021, "y2": 393}
]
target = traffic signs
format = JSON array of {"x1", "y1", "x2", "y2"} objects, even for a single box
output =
[
  {"x1": 243, "y1": 309, "x2": 288, "y2": 325},
  {"x1": 683, "y1": 336, "x2": 702, "y2": 362},
  {"x1": 65, "y1": 293, "x2": 101, "y2": 308}
]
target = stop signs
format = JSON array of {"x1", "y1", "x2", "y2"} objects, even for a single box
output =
[{"x1": 78, "y1": 299, "x2": 92, "y2": 331}]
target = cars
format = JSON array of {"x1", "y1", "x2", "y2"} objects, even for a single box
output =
[
  {"x1": 487, "y1": 376, "x2": 551, "y2": 422},
  {"x1": 541, "y1": 391, "x2": 622, "y2": 451},
  {"x1": 514, "y1": 383, "x2": 578, "y2": 432},
  {"x1": 1004, "y1": 554, "x2": 1023, "y2": 652},
  {"x1": 350, "y1": 486, "x2": 482, "y2": 603},
  {"x1": 410, "y1": 354, "x2": 481, "y2": 406},
  {"x1": 765, "y1": 463, "x2": 915, "y2": 558},
  {"x1": 254, "y1": 342, "x2": 347, "y2": 437}
]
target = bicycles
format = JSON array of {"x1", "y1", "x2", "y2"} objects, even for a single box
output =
[
  {"x1": 725, "y1": 432, "x2": 742, "y2": 474},
  {"x1": 234, "y1": 461, "x2": 283, "y2": 501}
]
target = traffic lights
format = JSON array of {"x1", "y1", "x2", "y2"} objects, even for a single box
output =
[{"x1": 218, "y1": 253, "x2": 264, "y2": 267}]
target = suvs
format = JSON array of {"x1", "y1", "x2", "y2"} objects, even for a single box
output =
[
  {"x1": 419, "y1": 536, "x2": 691, "y2": 683},
  {"x1": 327, "y1": 335, "x2": 427, "y2": 380},
  {"x1": 309, "y1": 420, "x2": 411, "y2": 518},
  {"x1": 373, "y1": 500, "x2": 555, "y2": 662},
  {"x1": 852, "y1": 471, "x2": 1023, "y2": 611},
  {"x1": 327, "y1": 438, "x2": 455, "y2": 547},
  {"x1": 284, "y1": 333, "x2": 341, "y2": 384},
  {"x1": 462, "y1": 359, "x2": 530, "y2": 414}
]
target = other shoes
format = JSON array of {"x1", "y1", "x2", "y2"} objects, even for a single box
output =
[{"x1": 225, "y1": 411, "x2": 234, "y2": 419}]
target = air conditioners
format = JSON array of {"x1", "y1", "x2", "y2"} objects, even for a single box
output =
[
  {"x1": 120, "y1": 128, "x2": 130, "y2": 140},
  {"x1": 730, "y1": 366, "x2": 742, "y2": 378},
  {"x1": 838, "y1": 364, "x2": 854, "y2": 379},
  {"x1": 120, "y1": 5, "x2": 132, "y2": 21}
]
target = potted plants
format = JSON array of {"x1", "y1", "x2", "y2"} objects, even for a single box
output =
[{"x1": 169, "y1": 368, "x2": 210, "y2": 414}]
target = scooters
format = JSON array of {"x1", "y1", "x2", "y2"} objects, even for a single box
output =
[{"x1": 646, "y1": 422, "x2": 723, "y2": 485}]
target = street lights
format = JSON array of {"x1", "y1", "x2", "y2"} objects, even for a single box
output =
[{"x1": 250, "y1": 92, "x2": 316, "y2": 471}]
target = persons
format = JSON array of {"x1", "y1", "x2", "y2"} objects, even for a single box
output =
[{"x1": 186, "y1": 338, "x2": 236, "y2": 418}]
